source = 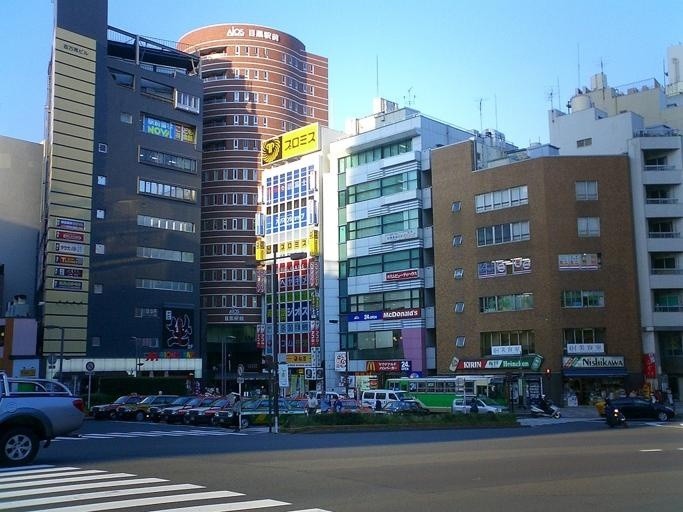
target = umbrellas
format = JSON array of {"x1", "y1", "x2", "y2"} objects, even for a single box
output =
[{"x1": 226, "y1": 392, "x2": 243, "y2": 405}]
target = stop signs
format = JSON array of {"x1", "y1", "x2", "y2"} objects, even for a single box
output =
[{"x1": 86, "y1": 362, "x2": 94, "y2": 371}]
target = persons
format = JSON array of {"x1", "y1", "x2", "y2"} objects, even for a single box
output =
[
  {"x1": 471, "y1": 396, "x2": 479, "y2": 412},
  {"x1": 232, "y1": 395, "x2": 242, "y2": 432},
  {"x1": 320, "y1": 391, "x2": 330, "y2": 412},
  {"x1": 603, "y1": 396, "x2": 615, "y2": 414},
  {"x1": 305, "y1": 393, "x2": 318, "y2": 414},
  {"x1": 540, "y1": 394, "x2": 553, "y2": 413},
  {"x1": 333, "y1": 395, "x2": 342, "y2": 412}
]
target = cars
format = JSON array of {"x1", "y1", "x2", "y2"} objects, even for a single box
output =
[{"x1": 609, "y1": 397, "x2": 675, "y2": 421}]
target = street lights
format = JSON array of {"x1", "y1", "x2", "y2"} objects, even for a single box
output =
[
  {"x1": 131, "y1": 336, "x2": 137, "y2": 377},
  {"x1": 44, "y1": 324, "x2": 64, "y2": 382},
  {"x1": 225, "y1": 336, "x2": 236, "y2": 395},
  {"x1": 247, "y1": 243, "x2": 307, "y2": 426}
]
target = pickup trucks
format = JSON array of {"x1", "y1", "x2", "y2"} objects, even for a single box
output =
[{"x1": 0, "y1": 370, "x2": 86, "y2": 467}]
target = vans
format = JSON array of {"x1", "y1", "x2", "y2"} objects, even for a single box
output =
[{"x1": 451, "y1": 397, "x2": 510, "y2": 415}]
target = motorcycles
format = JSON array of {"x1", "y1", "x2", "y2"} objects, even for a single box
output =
[
  {"x1": 529, "y1": 402, "x2": 561, "y2": 419},
  {"x1": 604, "y1": 399, "x2": 627, "y2": 428}
]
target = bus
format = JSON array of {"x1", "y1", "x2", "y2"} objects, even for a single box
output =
[{"x1": 385, "y1": 375, "x2": 509, "y2": 412}]
